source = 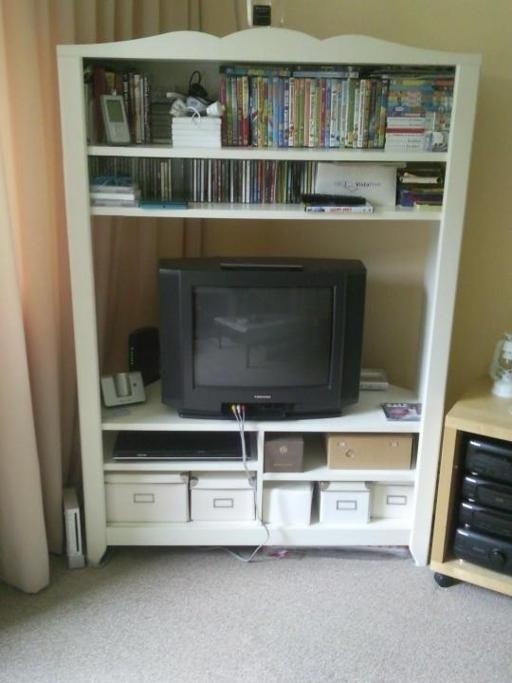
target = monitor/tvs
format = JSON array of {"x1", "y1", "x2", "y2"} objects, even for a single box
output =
[{"x1": 157, "y1": 255, "x2": 366, "y2": 420}]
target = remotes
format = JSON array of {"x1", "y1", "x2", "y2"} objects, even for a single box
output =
[{"x1": 300, "y1": 191, "x2": 365, "y2": 205}]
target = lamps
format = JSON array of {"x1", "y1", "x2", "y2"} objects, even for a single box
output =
[{"x1": 489, "y1": 330, "x2": 512, "y2": 398}]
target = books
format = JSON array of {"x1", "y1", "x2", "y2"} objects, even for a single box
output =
[
  {"x1": 358, "y1": 366, "x2": 391, "y2": 391},
  {"x1": 379, "y1": 400, "x2": 423, "y2": 421},
  {"x1": 83, "y1": 62, "x2": 151, "y2": 145},
  {"x1": 222, "y1": 63, "x2": 454, "y2": 151},
  {"x1": 85, "y1": 155, "x2": 446, "y2": 214}
]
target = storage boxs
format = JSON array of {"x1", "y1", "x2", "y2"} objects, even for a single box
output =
[
  {"x1": 323, "y1": 433, "x2": 414, "y2": 470},
  {"x1": 103, "y1": 472, "x2": 413, "y2": 523}
]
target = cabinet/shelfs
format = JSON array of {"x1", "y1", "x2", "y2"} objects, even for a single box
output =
[
  {"x1": 54, "y1": 26, "x2": 483, "y2": 567},
  {"x1": 428, "y1": 387, "x2": 512, "y2": 597}
]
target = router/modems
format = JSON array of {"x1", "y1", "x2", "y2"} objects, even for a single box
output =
[{"x1": 62, "y1": 486, "x2": 86, "y2": 570}]
